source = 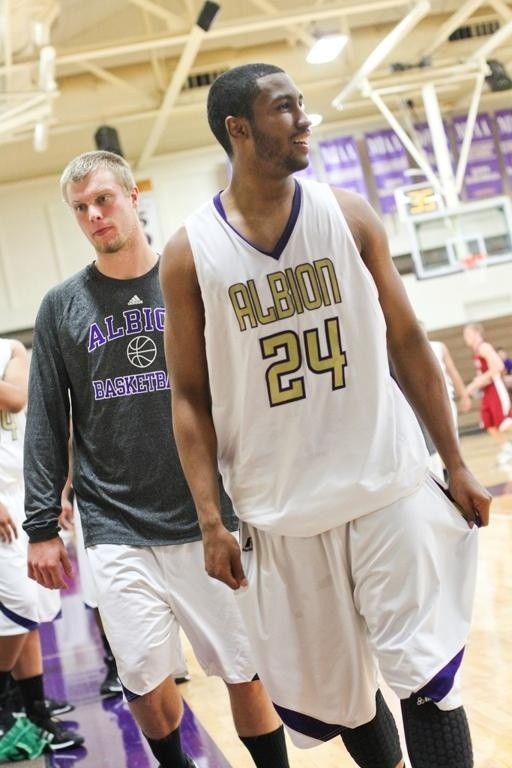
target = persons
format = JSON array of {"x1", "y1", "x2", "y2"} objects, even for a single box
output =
[
  {"x1": 158, "y1": 65, "x2": 492, "y2": 768},
  {"x1": 414, "y1": 320, "x2": 512, "y2": 483},
  {"x1": 21, "y1": 151, "x2": 289, "y2": 768}
]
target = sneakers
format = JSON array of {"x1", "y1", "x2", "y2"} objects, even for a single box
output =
[
  {"x1": 32, "y1": 694, "x2": 75, "y2": 717},
  {"x1": 27, "y1": 711, "x2": 85, "y2": 753},
  {"x1": 96, "y1": 655, "x2": 123, "y2": 700}
]
